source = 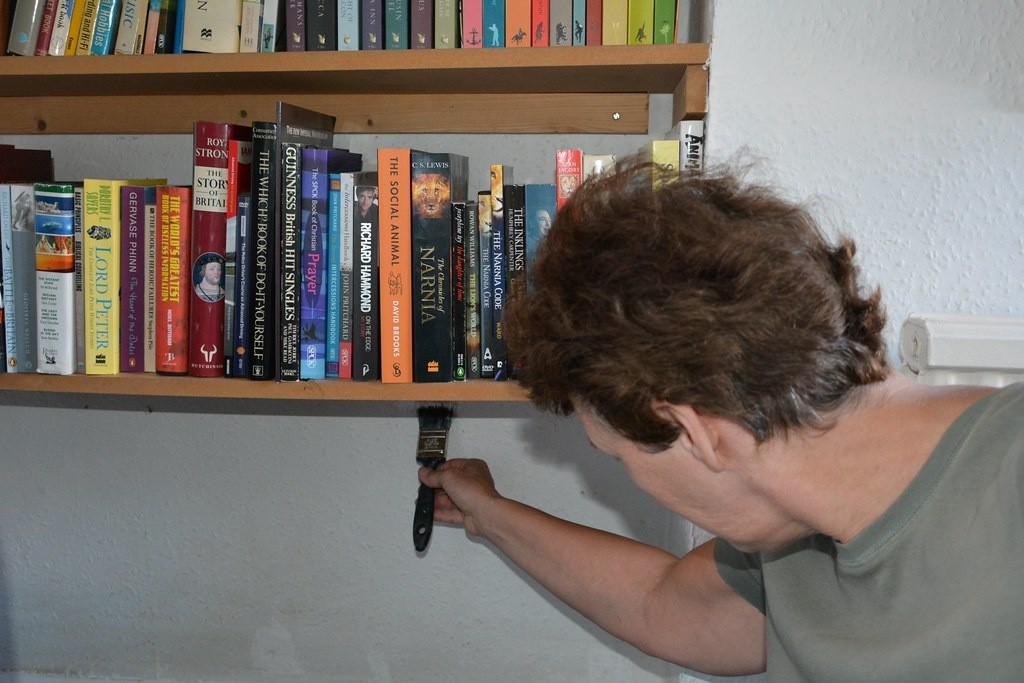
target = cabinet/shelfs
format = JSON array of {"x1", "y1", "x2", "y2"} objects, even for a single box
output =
[{"x1": 1, "y1": 0, "x2": 710, "y2": 400}]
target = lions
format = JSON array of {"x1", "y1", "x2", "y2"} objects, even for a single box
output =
[{"x1": 412, "y1": 174, "x2": 450, "y2": 218}]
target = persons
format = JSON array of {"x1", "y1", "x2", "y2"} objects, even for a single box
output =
[{"x1": 417, "y1": 146, "x2": 1024, "y2": 683}]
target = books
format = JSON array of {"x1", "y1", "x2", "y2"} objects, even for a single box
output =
[
  {"x1": 1, "y1": 96, "x2": 706, "y2": 384},
  {"x1": 0, "y1": 0, "x2": 714, "y2": 55}
]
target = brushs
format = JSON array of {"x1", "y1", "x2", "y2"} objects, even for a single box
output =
[{"x1": 412, "y1": 402, "x2": 459, "y2": 553}]
